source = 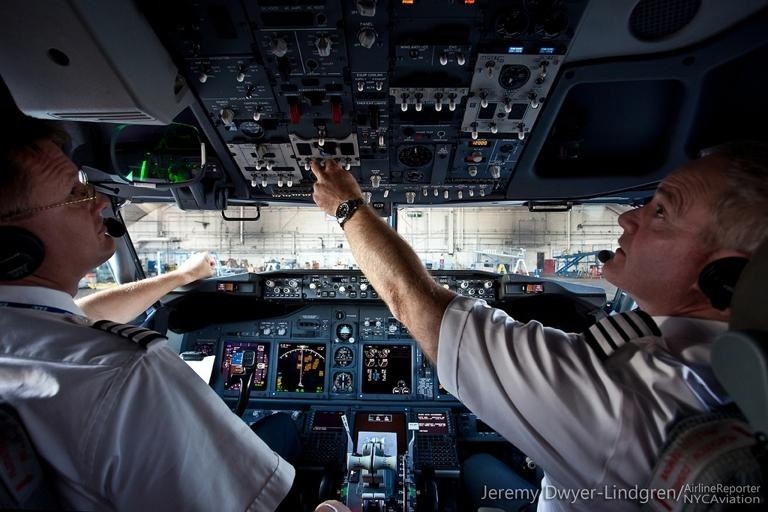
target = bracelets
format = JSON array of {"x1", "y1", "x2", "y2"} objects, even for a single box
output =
[{"x1": 314, "y1": 502, "x2": 339, "y2": 512}]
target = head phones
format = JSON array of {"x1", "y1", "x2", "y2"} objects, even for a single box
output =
[
  {"x1": 0, "y1": 226, "x2": 46, "y2": 280},
  {"x1": 698, "y1": 256, "x2": 750, "y2": 312}
]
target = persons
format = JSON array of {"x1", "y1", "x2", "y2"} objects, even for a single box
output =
[
  {"x1": 308, "y1": 133, "x2": 766, "y2": 510},
  {"x1": 1, "y1": 109, "x2": 350, "y2": 511}
]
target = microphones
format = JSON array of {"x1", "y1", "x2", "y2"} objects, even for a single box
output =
[
  {"x1": 102, "y1": 217, "x2": 126, "y2": 238},
  {"x1": 598, "y1": 250, "x2": 615, "y2": 263}
]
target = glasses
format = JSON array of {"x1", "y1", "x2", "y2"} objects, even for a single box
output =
[{"x1": 0, "y1": 170, "x2": 95, "y2": 222}]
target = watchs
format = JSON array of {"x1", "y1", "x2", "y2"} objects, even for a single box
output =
[{"x1": 335, "y1": 197, "x2": 367, "y2": 230}]
